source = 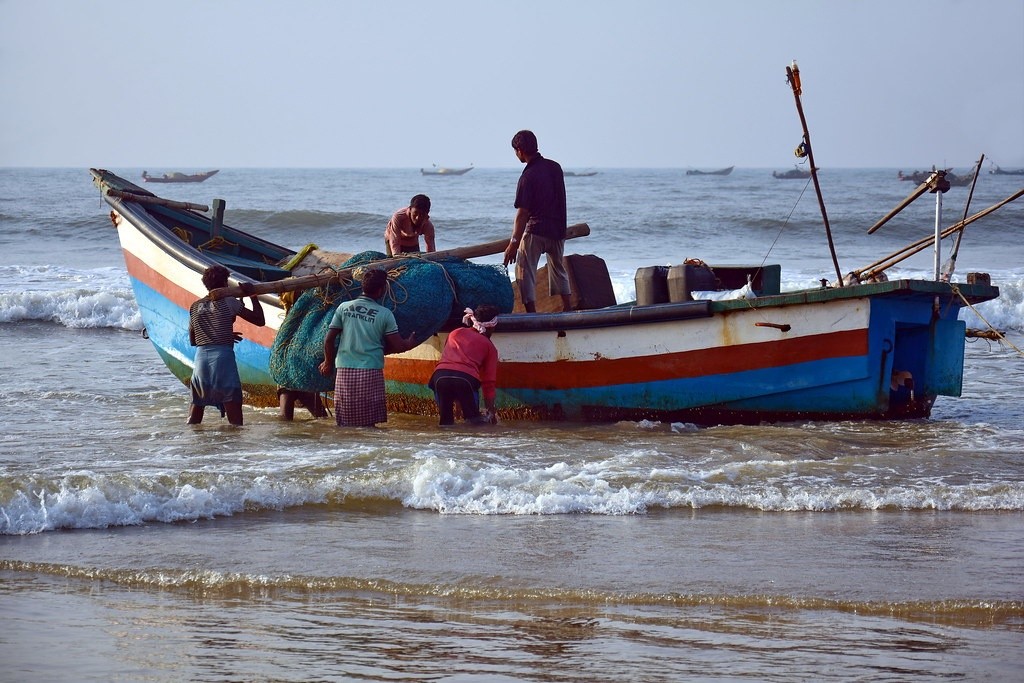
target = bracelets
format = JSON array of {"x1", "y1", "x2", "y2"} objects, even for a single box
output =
[{"x1": 249, "y1": 294, "x2": 258, "y2": 298}]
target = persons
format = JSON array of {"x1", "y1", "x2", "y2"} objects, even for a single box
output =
[
  {"x1": 186, "y1": 266, "x2": 266, "y2": 427},
  {"x1": 383, "y1": 194, "x2": 435, "y2": 258},
  {"x1": 317, "y1": 269, "x2": 415, "y2": 428},
  {"x1": 428, "y1": 304, "x2": 499, "y2": 427},
  {"x1": 503, "y1": 130, "x2": 571, "y2": 315},
  {"x1": 276, "y1": 291, "x2": 329, "y2": 419}
]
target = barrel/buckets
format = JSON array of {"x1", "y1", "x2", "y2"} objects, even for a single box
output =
[
  {"x1": 635, "y1": 265, "x2": 673, "y2": 307},
  {"x1": 667, "y1": 263, "x2": 717, "y2": 303}
]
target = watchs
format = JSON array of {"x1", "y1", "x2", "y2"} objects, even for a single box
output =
[{"x1": 510, "y1": 237, "x2": 520, "y2": 244}]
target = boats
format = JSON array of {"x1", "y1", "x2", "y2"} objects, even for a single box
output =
[
  {"x1": 687, "y1": 165, "x2": 735, "y2": 177},
  {"x1": 899, "y1": 153, "x2": 1024, "y2": 188},
  {"x1": 412, "y1": 163, "x2": 477, "y2": 179},
  {"x1": 141, "y1": 169, "x2": 221, "y2": 184},
  {"x1": 562, "y1": 171, "x2": 600, "y2": 179},
  {"x1": 89, "y1": 55, "x2": 1002, "y2": 430},
  {"x1": 772, "y1": 165, "x2": 813, "y2": 179}
]
